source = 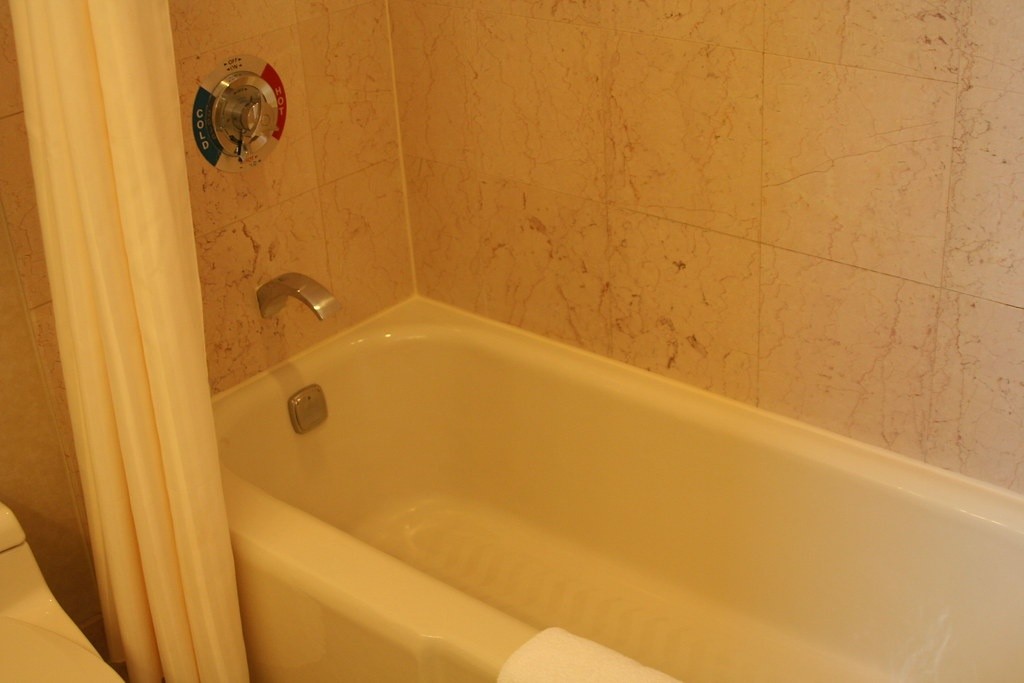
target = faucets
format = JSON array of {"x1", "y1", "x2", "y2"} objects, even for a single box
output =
[{"x1": 257, "y1": 269, "x2": 343, "y2": 320}]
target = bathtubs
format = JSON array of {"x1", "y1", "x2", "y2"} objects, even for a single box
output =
[{"x1": 207, "y1": 293, "x2": 1024, "y2": 683}]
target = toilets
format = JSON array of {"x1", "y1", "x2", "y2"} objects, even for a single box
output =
[{"x1": 0, "y1": 500, "x2": 127, "y2": 683}]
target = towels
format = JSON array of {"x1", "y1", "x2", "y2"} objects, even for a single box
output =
[{"x1": 494, "y1": 622, "x2": 677, "y2": 683}]
